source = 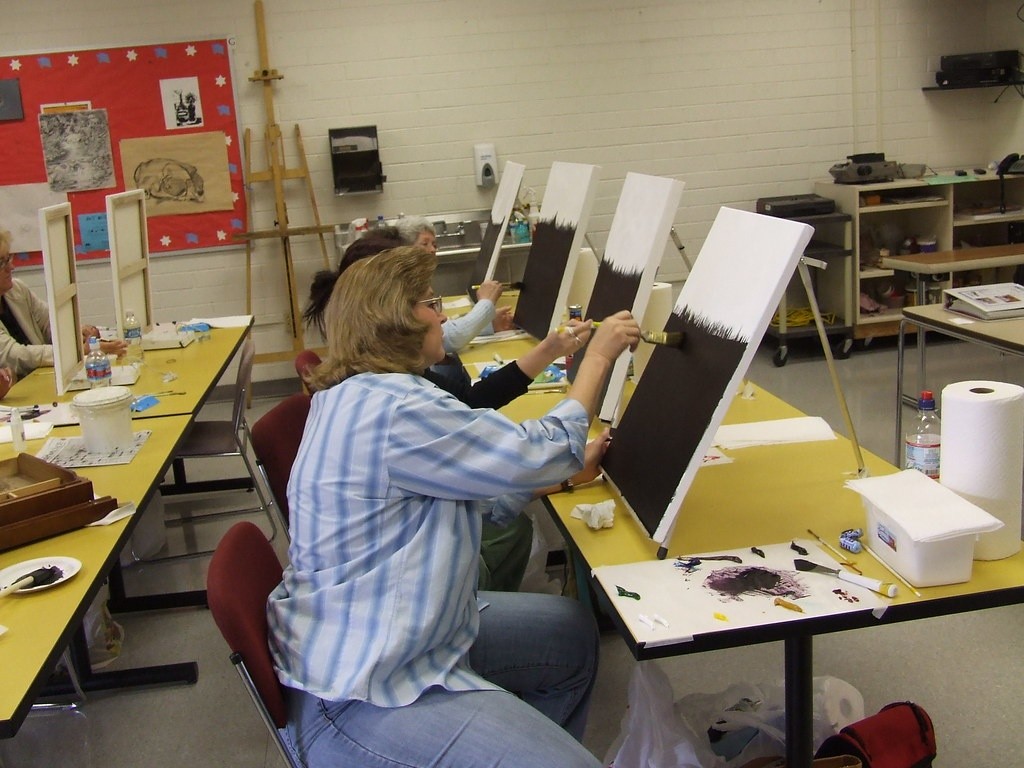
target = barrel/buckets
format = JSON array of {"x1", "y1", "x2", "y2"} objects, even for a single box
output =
[{"x1": 69, "y1": 386, "x2": 138, "y2": 453}]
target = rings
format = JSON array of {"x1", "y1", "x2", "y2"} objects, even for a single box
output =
[
  {"x1": 575, "y1": 335, "x2": 582, "y2": 343},
  {"x1": 568, "y1": 327, "x2": 575, "y2": 336}
]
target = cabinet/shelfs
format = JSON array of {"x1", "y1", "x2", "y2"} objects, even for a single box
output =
[
  {"x1": 765, "y1": 211, "x2": 854, "y2": 367},
  {"x1": 814, "y1": 172, "x2": 1023, "y2": 340}
]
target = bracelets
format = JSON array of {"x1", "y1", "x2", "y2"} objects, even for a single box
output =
[{"x1": 561, "y1": 478, "x2": 573, "y2": 492}]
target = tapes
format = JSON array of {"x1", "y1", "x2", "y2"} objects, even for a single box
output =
[
  {"x1": 877, "y1": 282, "x2": 892, "y2": 296},
  {"x1": 883, "y1": 286, "x2": 896, "y2": 298}
]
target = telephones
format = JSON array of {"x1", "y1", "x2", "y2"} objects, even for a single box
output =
[{"x1": 996, "y1": 153, "x2": 1024, "y2": 176}]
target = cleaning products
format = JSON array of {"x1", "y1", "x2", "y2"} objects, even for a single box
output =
[
  {"x1": 351, "y1": 217, "x2": 368, "y2": 241},
  {"x1": 513, "y1": 187, "x2": 541, "y2": 244}
]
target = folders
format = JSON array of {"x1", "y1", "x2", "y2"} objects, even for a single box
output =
[{"x1": 942, "y1": 282, "x2": 1024, "y2": 322}]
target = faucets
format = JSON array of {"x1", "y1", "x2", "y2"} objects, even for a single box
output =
[{"x1": 456, "y1": 220, "x2": 473, "y2": 236}]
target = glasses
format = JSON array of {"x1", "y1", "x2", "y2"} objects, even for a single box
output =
[
  {"x1": 0, "y1": 254, "x2": 13, "y2": 270},
  {"x1": 417, "y1": 295, "x2": 443, "y2": 313}
]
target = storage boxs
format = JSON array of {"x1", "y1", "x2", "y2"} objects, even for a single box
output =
[{"x1": 860, "y1": 495, "x2": 975, "y2": 589}]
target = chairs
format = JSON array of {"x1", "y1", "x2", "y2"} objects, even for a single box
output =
[
  {"x1": 295, "y1": 348, "x2": 322, "y2": 398},
  {"x1": 127, "y1": 336, "x2": 277, "y2": 567},
  {"x1": 251, "y1": 395, "x2": 312, "y2": 530},
  {"x1": 208, "y1": 522, "x2": 296, "y2": 768}
]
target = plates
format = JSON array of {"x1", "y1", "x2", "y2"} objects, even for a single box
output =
[{"x1": 0, "y1": 556, "x2": 83, "y2": 594}]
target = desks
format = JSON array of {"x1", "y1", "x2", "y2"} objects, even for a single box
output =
[
  {"x1": 437, "y1": 288, "x2": 1024, "y2": 767},
  {"x1": 0, "y1": 315, "x2": 255, "y2": 741},
  {"x1": 883, "y1": 243, "x2": 1023, "y2": 417},
  {"x1": 894, "y1": 303, "x2": 1023, "y2": 474}
]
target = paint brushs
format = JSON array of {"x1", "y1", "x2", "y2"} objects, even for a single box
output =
[
  {"x1": 472, "y1": 282, "x2": 528, "y2": 290},
  {"x1": 556, "y1": 326, "x2": 596, "y2": 334},
  {"x1": 807, "y1": 529, "x2": 850, "y2": 564}
]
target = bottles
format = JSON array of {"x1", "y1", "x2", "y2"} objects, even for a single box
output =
[
  {"x1": 374, "y1": 215, "x2": 389, "y2": 229},
  {"x1": 85, "y1": 336, "x2": 112, "y2": 389},
  {"x1": 565, "y1": 304, "x2": 583, "y2": 375},
  {"x1": 121, "y1": 311, "x2": 144, "y2": 360},
  {"x1": 10, "y1": 407, "x2": 26, "y2": 452},
  {"x1": 905, "y1": 390, "x2": 941, "y2": 483}
]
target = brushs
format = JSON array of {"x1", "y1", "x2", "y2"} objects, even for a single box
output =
[{"x1": 590, "y1": 322, "x2": 688, "y2": 349}]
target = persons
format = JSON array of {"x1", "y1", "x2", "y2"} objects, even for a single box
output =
[
  {"x1": 266, "y1": 248, "x2": 641, "y2": 768},
  {"x1": 399, "y1": 216, "x2": 517, "y2": 353},
  {"x1": 0, "y1": 230, "x2": 128, "y2": 398},
  {"x1": 304, "y1": 227, "x2": 594, "y2": 590}
]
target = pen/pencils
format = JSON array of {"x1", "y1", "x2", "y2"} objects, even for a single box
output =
[{"x1": 860, "y1": 540, "x2": 921, "y2": 598}]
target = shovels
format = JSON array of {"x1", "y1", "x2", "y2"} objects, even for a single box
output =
[{"x1": 794, "y1": 558, "x2": 897, "y2": 598}]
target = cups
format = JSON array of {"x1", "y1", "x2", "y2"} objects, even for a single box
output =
[
  {"x1": 917, "y1": 241, "x2": 937, "y2": 253},
  {"x1": 929, "y1": 287, "x2": 941, "y2": 304}
]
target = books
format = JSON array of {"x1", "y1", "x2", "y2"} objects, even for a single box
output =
[
  {"x1": 528, "y1": 365, "x2": 566, "y2": 389},
  {"x1": 943, "y1": 283, "x2": 1024, "y2": 322}
]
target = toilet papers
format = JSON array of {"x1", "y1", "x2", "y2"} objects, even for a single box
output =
[
  {"x1": 566, "y1": 247, "x2": 599, "y2": 322},
  {"x1": 631, "y1": 281, "x2": 673, "y2": 383},
  {"x1": 939, "y1": 379, "x2": 1024, "y2": 561}
]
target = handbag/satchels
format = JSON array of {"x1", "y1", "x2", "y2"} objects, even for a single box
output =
[{"x1": 604, "y1": 658, "x2": 936, "y2": 768}]
